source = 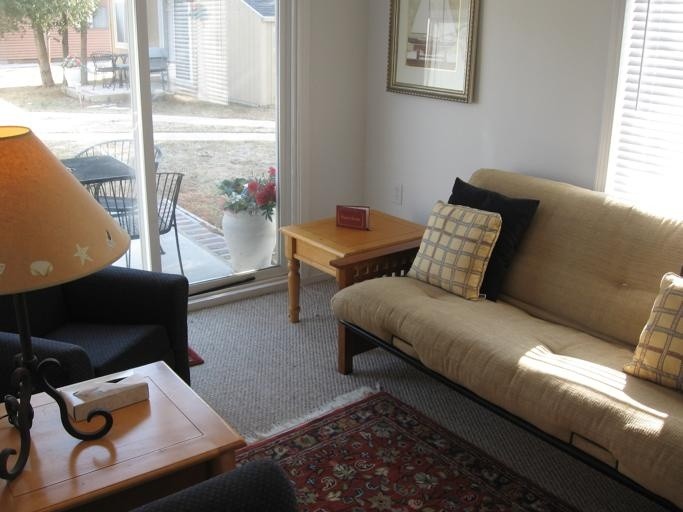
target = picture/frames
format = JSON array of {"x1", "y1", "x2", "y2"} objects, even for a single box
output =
[{"x1": 383, "y1": 0, "x2": 480, "y2": 103}]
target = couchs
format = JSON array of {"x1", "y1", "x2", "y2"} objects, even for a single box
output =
[
  {"x1": 327, "y1": 167, "x2": 683, "y2": 510},
  {"x1": 135, "y1": 458, "x2": 295, "y2": 512}
]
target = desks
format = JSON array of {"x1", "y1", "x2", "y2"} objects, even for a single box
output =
[
  {"x1": 280, "y1": 209, "x2": 426, "y2": 377},
  {"x1": 59, "y1": 157, "x2": 136, "y2": 200},
  {"x1": 2, "y1": 358, "x2": 245, "y2": 510}
]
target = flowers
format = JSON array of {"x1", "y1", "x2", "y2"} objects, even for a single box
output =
[
  {"x1": 60, "y1": 53, "x2": 81, "y2": 68},
  {"x1": 220, "y1": 168, "x2": 280, "y2": 217}
]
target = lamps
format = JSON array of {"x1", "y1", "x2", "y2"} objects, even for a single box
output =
[{"x1": 0, "y1": 127, "x2": 132, "y2": 482}]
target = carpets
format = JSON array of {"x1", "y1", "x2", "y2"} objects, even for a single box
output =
[{"x1": 231, "y1": 388, "x2": 576, "y2": 512}]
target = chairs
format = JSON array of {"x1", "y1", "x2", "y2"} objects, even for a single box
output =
[
  {"x1": 95, "y1": 168, "x2": 187, "y2": 280},
  {"x1": 74, "y1": 137, "x2": 164, "y2": 209},
  {"x1": 91, "y1": 42, "x2": 169, "y2": 92},
  {"x1": 0, "y1": 270, "x2": 192, "y2": 400}
]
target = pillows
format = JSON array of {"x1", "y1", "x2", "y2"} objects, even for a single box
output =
[
  {"x1": 404, "y1": 199, "x2": 499, "y2": 302},
  {"x1": 621, "y1": 267, "x2": 683, "y2": 394},
  {"x1": 442, "y1": 176, "x2": 539, "y2": 303}
]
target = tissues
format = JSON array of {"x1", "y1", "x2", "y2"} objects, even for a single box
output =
[{"x1": 57, "y1": 366, "x2": 150, "y2": 424}]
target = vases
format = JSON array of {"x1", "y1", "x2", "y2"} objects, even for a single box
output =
[
  {"x1": 218, "y1": 206, "x2": 278, "y2": 269},
  {"x1": 63, "y1": 67, "x2": 81, "y2": 88}
]
